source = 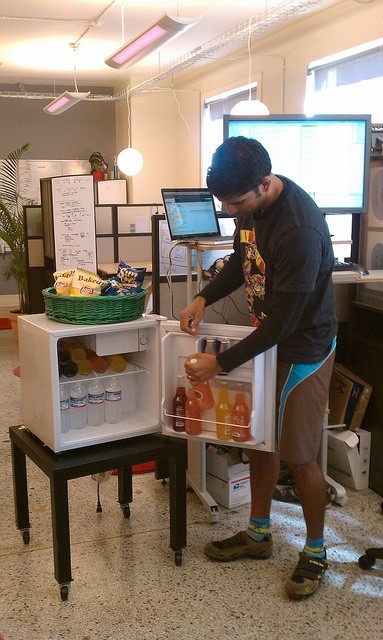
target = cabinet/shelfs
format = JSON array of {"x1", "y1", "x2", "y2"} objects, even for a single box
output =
[
  {"x1": 177, "y1": 239, "x2": 383, "y2": 323},
  {"x1": 23, "y1": 202, "x2": 165, "y2": 315},
  {"x1": 346, "y1": 304, "x2": 383, "y2": 423}
]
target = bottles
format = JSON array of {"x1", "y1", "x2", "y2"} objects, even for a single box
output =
[
  {"x1": 57, "y1": 341, "x2": 127, "y2": 377},
  {"x1": 87, "y1": 379, "x2": 105, "y2": 426},
  {"x1": 59, "y1": 384, "x2": 69, "y2": 434},
  {"x1": 185, "y1": 376, "x2": 202, "y2": 435},
  {"x1": 69, "y1": 381, "x2": 87, "y2": 429},
  {"x1": 219, "y1": 341, "x2": 230, "y2": 376},
  {"x1": 232, "y1": 382, "x2": 250, "y2": 442},
  {"x1": 172, "y1": 375, "x2": 188, "y2": 432},
  {"x1": 190, "y1": 357, "x2": 215, "y2": 411},
  {"x1": 216, "y1": 381, "x2": 232, "y2": 440},
  {"x1": 205, "y1": 339, "x2": 217, "y2": 354},
  {"x1": 104, "y1": 376, "x2": 122, "y2": 424}
]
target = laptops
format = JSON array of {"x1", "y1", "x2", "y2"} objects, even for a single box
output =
[{"x1": 158, "y1": 188, "x2": 234, "y2": 245}]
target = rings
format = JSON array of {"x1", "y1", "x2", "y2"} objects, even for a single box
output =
[{"x1": 186, "y1": 374, "x2": 193, "y2": 382}]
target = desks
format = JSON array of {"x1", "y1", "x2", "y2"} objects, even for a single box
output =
[
  {"x1": 9, "y1": 425, "x2": 188, "y2": 602},
  {"x1": 97, "y1": 260, "x2": 152, "y2": 281}
]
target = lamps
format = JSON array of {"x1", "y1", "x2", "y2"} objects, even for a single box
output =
[
  {"x1": 104, "y1": 2, "x2": 205, "y2": 70},
  {"x1": 230, "y1": 15, "x2": 270, "y2": 116},
  {"x1": 42, "y1": 46, "x2": 91, "y2": 116},
  {"x1": 116, "y1": 83, "x2": 144, "y2": 176}
]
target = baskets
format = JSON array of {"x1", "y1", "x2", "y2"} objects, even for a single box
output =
[{"x1": 42, "y1": 286, "x2": 146, "y2": 325}]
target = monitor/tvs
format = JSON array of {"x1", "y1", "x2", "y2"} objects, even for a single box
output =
[{"x1": 223, "y1": 113, "x2": 373, "y2": 214}]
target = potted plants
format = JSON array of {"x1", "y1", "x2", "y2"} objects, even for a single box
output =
[
  {"x1": 1, "y1": 141, "x2": 39, "y2": 349},
  {"x1": 88, "y1": 150, "x2": 108, "y2": 182}
]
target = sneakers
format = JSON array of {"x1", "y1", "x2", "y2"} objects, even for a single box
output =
[
  {"x1": 284, "y1": 546, "x2": 328, "y2": 597},
  {"x1": 203, "y1": 530, "x2": 274, "y2": 562}
]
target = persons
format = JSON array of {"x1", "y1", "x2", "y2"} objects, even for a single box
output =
[{"x1": 180, "y1": 136, "x2": 340, "y2": 600}]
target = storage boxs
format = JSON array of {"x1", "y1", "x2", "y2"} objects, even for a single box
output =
[
  {"x1": 206, "y1": 450, "x2": 252, "y2": 510},
  {"x1": 329, "y1": 362, "x2": 373, "y2": 431},
  {"x1": 327, "y1": 427, "x2": 372, "y2": 491}
]
